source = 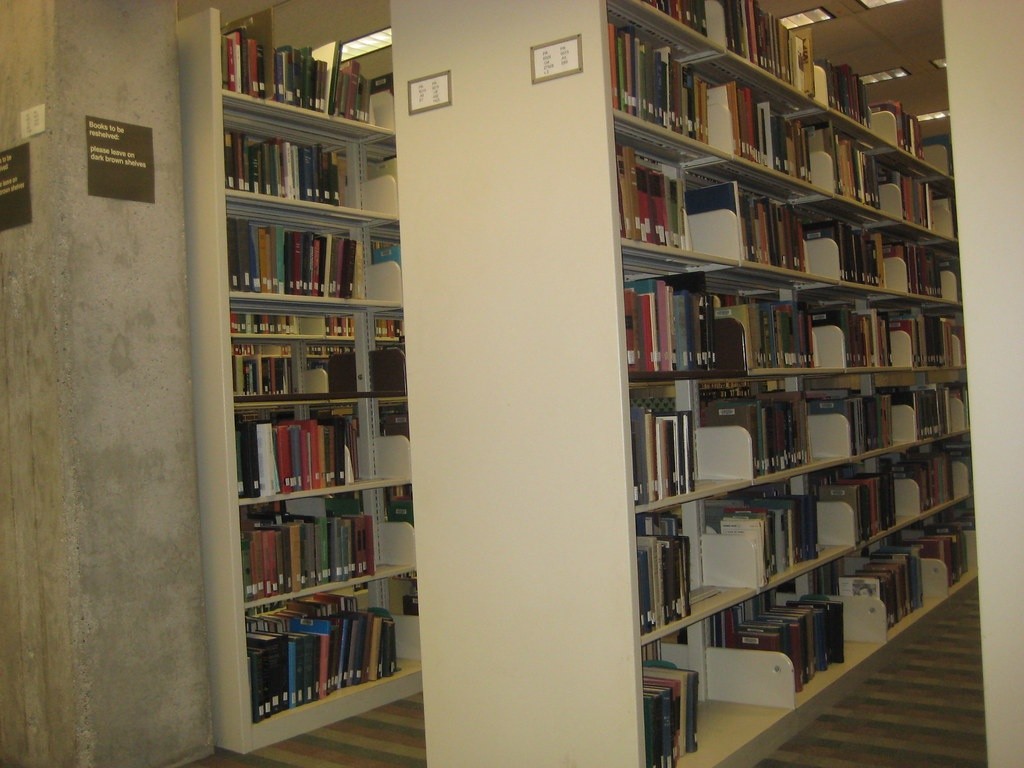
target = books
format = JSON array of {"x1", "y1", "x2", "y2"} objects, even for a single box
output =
[
  {"x1": 221, "y1": 29, "x2": 419, "y2": 724},
  {"x1": 607, "y1": 0, "x2": 975, "y2": 768}
]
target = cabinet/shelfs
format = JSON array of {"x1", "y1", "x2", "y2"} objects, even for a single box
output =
[
  {"x1": 391, "y1": 0, "x2": 980, "y2": 768},
  {"x1": 179, "y1": 8, "x2": 423, "y2": 755}
]
